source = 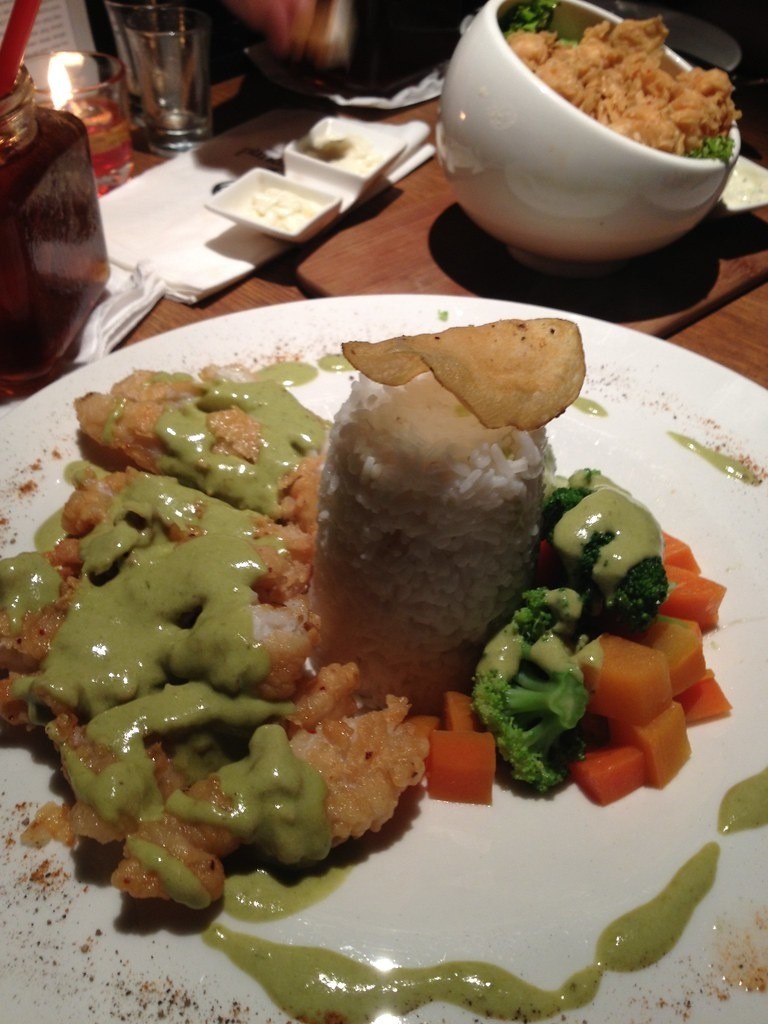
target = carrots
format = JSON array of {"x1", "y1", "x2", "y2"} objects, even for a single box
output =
[
  {"x1": 400, "y1": 689, "x2": 497, "y2": 808},
  {"x1": 566, "y1": 531, "x2": 725, "y2": 805}
]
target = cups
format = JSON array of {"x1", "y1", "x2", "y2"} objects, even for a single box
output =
[
  {"x1": 104, "y1": 0, "x2": 191, "y2": 107},
  {"x1": 22, "y1": 49, "x2": 133, "y2": 194},
  {"x1": 129, "y1": 18, "x2": 215, "y2": 154}
]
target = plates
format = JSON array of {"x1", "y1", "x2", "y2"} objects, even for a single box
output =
[
  {"x1": 205, "y1": 118, "x2": 407, "y2": 243},
  {"x1": 0, "y1": 292, "x2": 768, "y2": 1024},
  {"x1": 722, "y1": 155, "x2": 768, "y2": 213}
]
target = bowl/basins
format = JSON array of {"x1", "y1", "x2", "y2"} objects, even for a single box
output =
[{"x1": 430, "y1": 0, "x2": 743, "y2": 272}]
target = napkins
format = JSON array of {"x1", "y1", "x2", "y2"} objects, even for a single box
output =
[
  {"x1": 95, "y1": 103, "x2": 435, "y2": 306},
  {"x1": 72, "y1": 228, "x2": 164, "y2": 365}
]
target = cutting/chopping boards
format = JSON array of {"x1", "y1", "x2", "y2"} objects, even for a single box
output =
[{"x1": 270, "y1": 98, "x2": 768, "y2": 337}]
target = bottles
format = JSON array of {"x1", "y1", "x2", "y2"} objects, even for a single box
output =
[{"x1": 0, "y1": 63, "x2": 111, "y2": 403}]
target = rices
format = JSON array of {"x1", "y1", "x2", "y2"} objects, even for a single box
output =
[{"x1": 305, "y1": 364, "x2": 558, "y2": 716}]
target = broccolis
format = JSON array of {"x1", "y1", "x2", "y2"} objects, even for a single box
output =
[{"x1": 468, "y1": 463, "x2": 666, "y2": 798}]
